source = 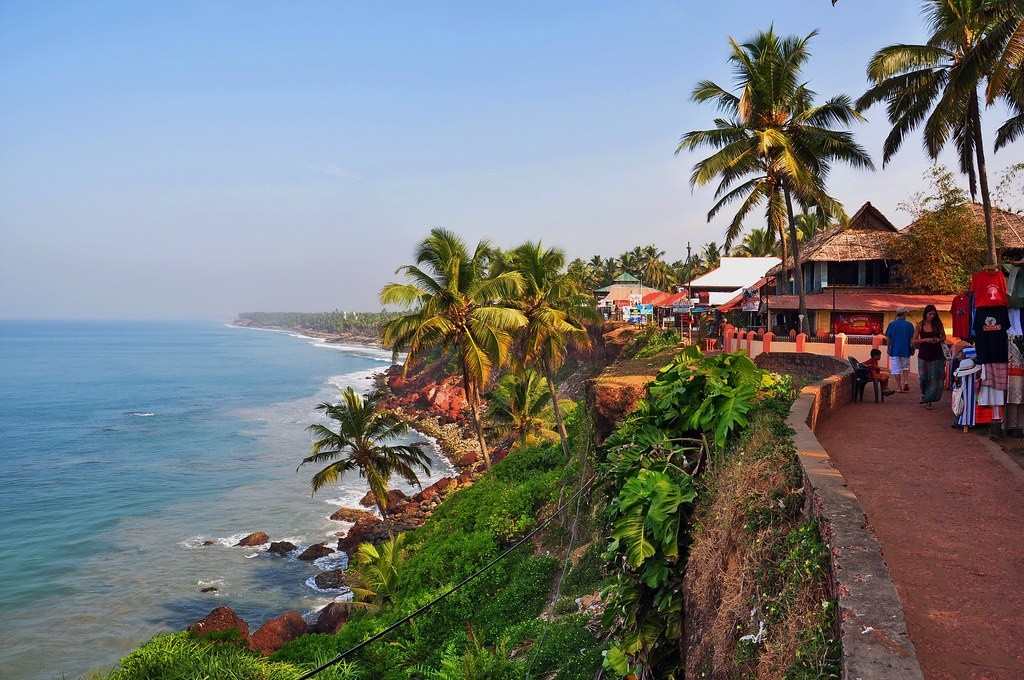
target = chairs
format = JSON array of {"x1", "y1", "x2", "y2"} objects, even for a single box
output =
[{"x1": 848, "y1": 355, "x2": 885, "y2": 405}]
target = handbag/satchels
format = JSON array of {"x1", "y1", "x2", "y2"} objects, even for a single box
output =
[
  {"x1": 951, "y1": 388, "x2": 964, "y2": 417},
  {"x1": 940, "y1": 343, "x2": 952, "y2": 361}
]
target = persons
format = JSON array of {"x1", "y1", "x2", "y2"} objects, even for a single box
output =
[
  {"x1": 885, "y1": 308, "x2": 915, "y2": 393},
  {"x1": 860, "y1": 349, "x2": 895, "y2": 397},
  {"x1": 912, "y1": 305, "x2": 946, "y2": 410}
]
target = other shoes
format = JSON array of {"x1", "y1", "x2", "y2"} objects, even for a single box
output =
[
  {"x1": 896, "y1": 389, "x2": 902, "y2": 393},
  {"x1": 881, "y1": 389, "x2": 895, "y2": 397},
  {"x1": 903, "y1": 381, "x2": 909, "y2": 392}
]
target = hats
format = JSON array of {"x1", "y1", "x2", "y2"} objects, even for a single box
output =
[
  {"x1": 896, "y1": 307, "x2": 909, "y2": 315},
  {"x1": 953, "y1": 359, "x2": 981, "y2": 377}
]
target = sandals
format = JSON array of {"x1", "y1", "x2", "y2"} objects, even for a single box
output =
[
  {"x1": 926, "y1": 401, "x2": 933, "y2": 410},
  {"x1": 918, "y1": 394, "x2": 925, "y2": 404}
]
